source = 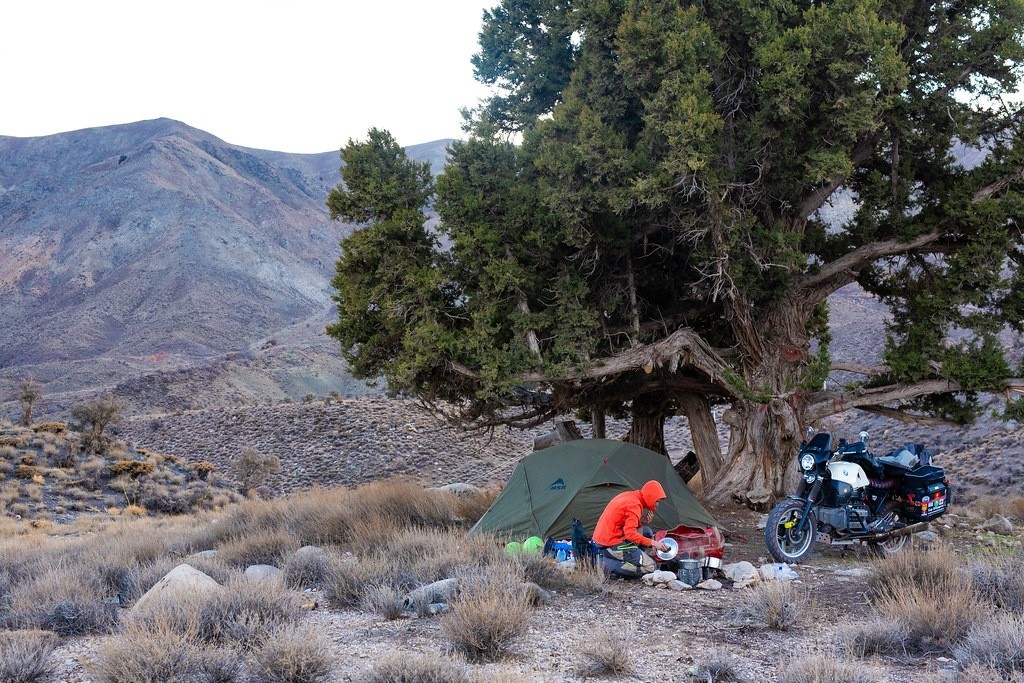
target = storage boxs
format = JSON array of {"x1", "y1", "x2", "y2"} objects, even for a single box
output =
[{"x1": 903, "y1": 481, "x2": 951, "y2": 521}]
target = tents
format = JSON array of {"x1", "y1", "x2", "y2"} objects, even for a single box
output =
[{"x1": 468, "y1": 438, "x2": 729, "y2": 549}]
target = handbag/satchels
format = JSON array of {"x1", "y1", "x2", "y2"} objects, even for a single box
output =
[{"x1": 652, "y1": 521, "x2": 725, "y2": 564}]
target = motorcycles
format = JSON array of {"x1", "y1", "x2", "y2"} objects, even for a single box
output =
[{"x1": 765, "y1": 427, "x2": 953, "y2": 564}]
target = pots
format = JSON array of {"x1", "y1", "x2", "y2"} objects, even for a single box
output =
[{"x1": 679, "y1": 560, "x2": 701, "y2": 569}]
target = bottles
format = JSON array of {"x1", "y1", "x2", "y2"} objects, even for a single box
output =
[
  {"x1": 556, "y1": 548, "x2": 566, "y2": 563},
  {"x1": 777, "y1": 567, "x2": 784, "y2": 582}
]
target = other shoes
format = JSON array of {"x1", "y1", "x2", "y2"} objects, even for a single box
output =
[{"x1": 596, "y1": 554, "x2": 609, "y2": 583}]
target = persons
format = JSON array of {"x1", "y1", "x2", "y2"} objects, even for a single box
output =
[{"x1": 590, "y1": 479, "x2": 668, "y2": 578}]
